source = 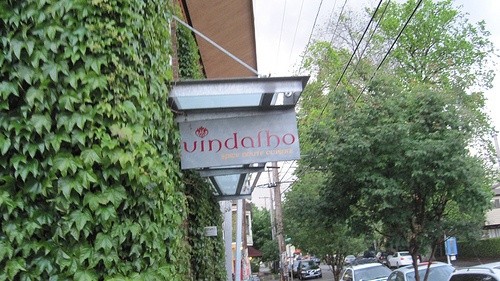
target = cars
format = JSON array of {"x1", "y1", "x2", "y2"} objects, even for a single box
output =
[
  {"x1": 387, "y1": 261, "x2": 456, "y2": 281},
  {"x1": 448, "y1": 262, "x2": 500, "y2": 281},
  {"x1": 286, "y1": 255, "x2": 322, "y2": 280},
  {"x1": 388, "y1": 251, "x2": 420, "y2": 268},
  {"x1": 325, "y1": 249, "x2": 389, "y2": 281},
  {"x1": 338, "y1": 262, "x2": 392, "y2": 281}
]
